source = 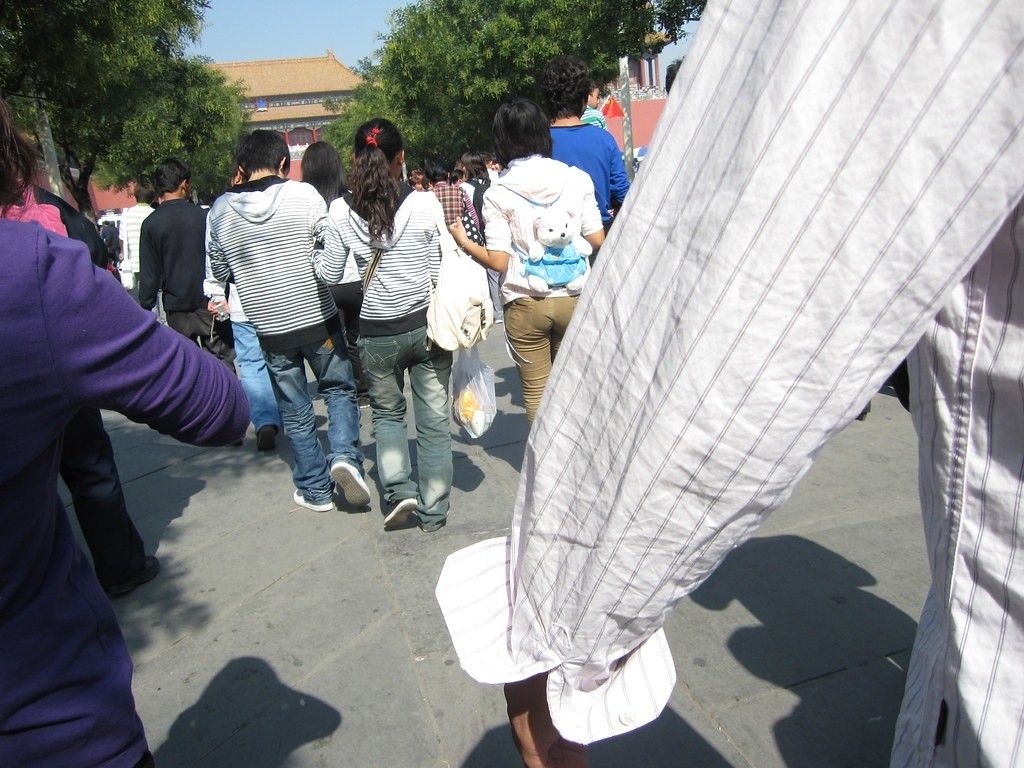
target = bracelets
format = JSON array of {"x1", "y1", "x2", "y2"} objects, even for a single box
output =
[{"x1": 461, "y1": 239, "x2": 469, "y2": 248}]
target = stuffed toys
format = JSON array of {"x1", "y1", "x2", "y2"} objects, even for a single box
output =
[{"x1": 527, "y1": 206, "x2": 593, "y2": 293}]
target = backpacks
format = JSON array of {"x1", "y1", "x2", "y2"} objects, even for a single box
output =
[
  {"x1": 511, "y1": 192, "x2": 592, "y2": 292},
  {"x1": 466, "y1": 179, "x2": 492, "y2": 235}
]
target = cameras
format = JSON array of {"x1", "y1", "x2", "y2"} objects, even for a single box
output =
[{"x1": 214, "y1": 303, "x2": 230, "y2": 322}]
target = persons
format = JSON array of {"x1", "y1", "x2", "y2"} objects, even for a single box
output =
[
  {"x1": 0, "y1": 109, "x2": 251, "y2": 768},
  {"x1": 435, "y1": 0, "x2": 1024, "y2": 768},
  {"x1": 423, "y1": 55, "x2": 872, "y2": 437},
  {"x1": 203, "y1": 117, "x2": 453, "y2": 534}
]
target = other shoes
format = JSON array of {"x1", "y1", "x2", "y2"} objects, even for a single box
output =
[
  {"x1": 384, "y1": 497, "x2": 418, "y2": 526},
  {"x1": 107, "y1": 556, "x2": 158, "y2": 595},
  {"x1": 257, "y1": 426, "x2": 276, "y2": 450}
]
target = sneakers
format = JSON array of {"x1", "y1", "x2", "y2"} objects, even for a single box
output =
[
  {"x1": 330, "y1": 461, "x2": 370, "y2": 507},
  {"x1": 294, "y1": 488, "x2": 333, "y2": 511}
]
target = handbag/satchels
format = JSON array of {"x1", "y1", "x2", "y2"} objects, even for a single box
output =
[
  {"x1": 450, "y1": 345, "x2": 497, "y2": 440},
  {"x1": 459, "y1": 186, "x2": 484, "y2": 245},
  {"x1": 423, "y1": 193, "x2": 494, "y2": 351}
]
